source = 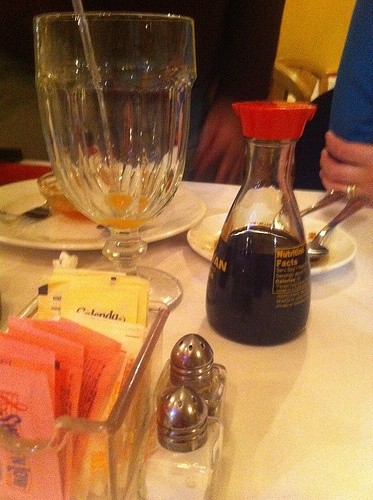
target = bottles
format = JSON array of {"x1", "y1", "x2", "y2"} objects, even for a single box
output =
[
  {"x1": 153, "y1": 335, "x2": 227, "y2": 421},
  {"x1": 206, "y1": 102, "x2": 316, "y2": 345},
  {"x1": 132, "y1": 386, "x2": 224, "y2": 500}
]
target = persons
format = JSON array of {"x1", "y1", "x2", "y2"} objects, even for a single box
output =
[
  {"x1": 2, "y1": 1, "x2": 284, "y2": 185},
  {"x1": 294, "y1": 0, "x2": 373, "y2": 205}
]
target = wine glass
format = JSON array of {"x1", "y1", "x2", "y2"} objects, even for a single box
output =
[{"x1": 34, "y1": 13, "x2": 197, "y2": 308}]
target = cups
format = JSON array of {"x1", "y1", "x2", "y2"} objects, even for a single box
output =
[{"x1": 37, "y1": 170, "x2": 96, "y2": 226}]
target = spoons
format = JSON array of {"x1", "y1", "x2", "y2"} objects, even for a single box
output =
[{"x1": 306, "y1": 196, "x2": 367, "y2": 260}]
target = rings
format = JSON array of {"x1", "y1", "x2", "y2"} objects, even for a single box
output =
[{"x1": 347, "y1": 186, "x2": 356, "y2": 200}]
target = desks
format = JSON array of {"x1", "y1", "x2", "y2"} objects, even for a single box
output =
[{"x1": 0, "y1": 179, "x2": 372, "y2": 498}]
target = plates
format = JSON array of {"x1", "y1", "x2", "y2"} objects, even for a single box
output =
[
  {"x1": 0, "y1": 175, "x2": 204, "y2": 250},
  {"x1": 186, "y1": 210, "x2": 356, "y2": 277}
]
